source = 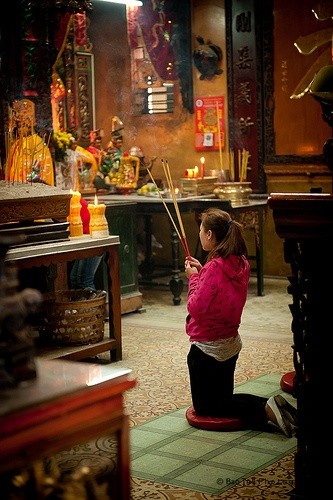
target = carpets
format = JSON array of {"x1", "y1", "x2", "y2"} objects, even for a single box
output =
[{"x1": 128, "y1": 373, "x2": 300, "y2": 495}]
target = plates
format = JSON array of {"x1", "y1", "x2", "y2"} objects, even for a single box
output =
[{"x1": 163, "y1": 192, "x2": 189, "y2": 198}]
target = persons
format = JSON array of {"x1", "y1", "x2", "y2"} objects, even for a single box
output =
[
  {"x1": 5, "y1": 99, "x2": 123, "y2": 196},
  {"x1": 184, "y1": 208, "x2": 297, "y2": 437}
]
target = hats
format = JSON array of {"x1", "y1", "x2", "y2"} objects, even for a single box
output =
[{"x1": 12, "y1": 99, "x2": 35, "y2": 126}]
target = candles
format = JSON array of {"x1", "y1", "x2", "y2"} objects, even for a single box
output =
[
  {"x1": 199, "y1": 156, "x2": 205, "y2": 179},
  {"x1": 68, "y1": 187, "x2": 84, "y2": 237},
  {"x1": 87, "y1": 192, "x2": 109, "y2": 239}
]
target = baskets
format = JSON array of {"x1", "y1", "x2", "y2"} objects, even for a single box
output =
[{"x1": 39, "y1": 289, "x2": 106, "y2": 346}]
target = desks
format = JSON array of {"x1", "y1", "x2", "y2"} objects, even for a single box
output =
[
  {"x1": 0, "y1": 181, "x2": 268, "y2": 500},
  {"x1": 268, "y1": 193, "x2": 333, "y2": 500}
]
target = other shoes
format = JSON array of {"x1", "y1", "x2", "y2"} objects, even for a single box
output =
[
  {"x1": 275, "y1": 395, "x2": 298, "y2": 427},
  {"x1": 265, "y1": 395, "x2": 292, "y2": 438}
]
